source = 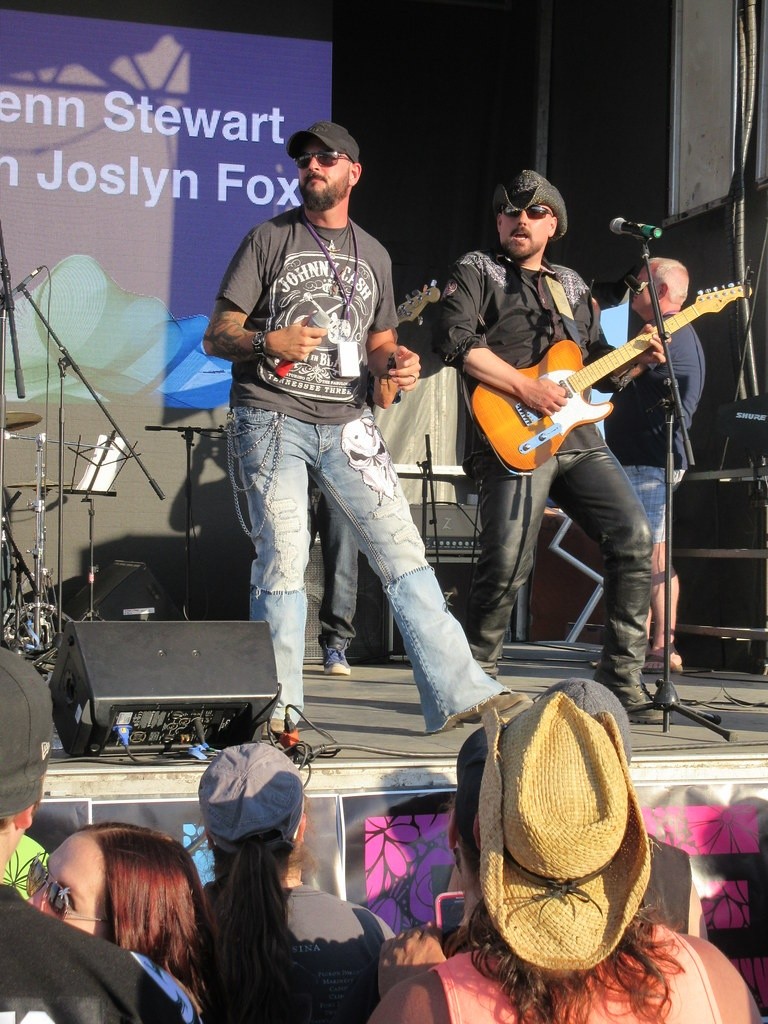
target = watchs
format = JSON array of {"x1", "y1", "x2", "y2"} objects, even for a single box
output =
[{"x1": 252, "y1": 328, "x2": 266, "y2": 359}]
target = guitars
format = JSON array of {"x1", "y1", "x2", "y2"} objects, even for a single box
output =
[
  {"x1": 471, "y1": 280, "x2": 754, "y2": 472},
  {"x1": 397, "y1": 280, "x2": 441, "y2": 327}
]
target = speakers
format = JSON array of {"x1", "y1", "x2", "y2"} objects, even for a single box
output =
[
  {"x1": 50, "y1": 559, "x2": 280, "y2": 755},
  {"x1": 354, "y1": 555, "x2": 480, "y2": 665}
]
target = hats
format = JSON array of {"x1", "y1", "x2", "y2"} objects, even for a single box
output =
[
  {"x1": 478, "y1": 679, "x2": 650, "y2": 969},
  {"x1": 493, "y1": 170, "x2": 567, "y2": 240},
  {"x1": 0, "y1": 647, "x2": 52, "y2": 819},
  {"x1": 198, "y1": 743, "x2": 305, "y2": 853},
  {"x1": 286, "y1": 119, "x2": 359, "y2": 162}
]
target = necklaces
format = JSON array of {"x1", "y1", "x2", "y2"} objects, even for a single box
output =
[{"x1": 304, "y1": 214, "x2": 352, "y2": 297}]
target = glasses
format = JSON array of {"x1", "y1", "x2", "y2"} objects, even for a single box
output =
[
  {"x1": 26, "y1": 858, "x2": 111, "y2": 924},
  {"x1": 501, "y1": 203, "x2": 553, "y2": 219},
  {"x1": 294, "y1": 147, "x2": 354, "y2": 169}
]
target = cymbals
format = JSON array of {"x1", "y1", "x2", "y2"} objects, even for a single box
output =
[
  {"x1": 6, "y1": 480, "x2": 78, "y2": 487},
  {"x1": 5, "y1": 411, "x2": 43, "y2": 433}
]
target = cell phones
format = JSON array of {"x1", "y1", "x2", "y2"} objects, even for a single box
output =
[{"x1": 436, "y1": 892, "x2": 466, "y2": 931}]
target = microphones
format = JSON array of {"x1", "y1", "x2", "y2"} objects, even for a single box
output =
[
  {"x1": 610, "y1": 217, "x2": 663, "y2": 238},
  {"x1": 11, "y1": 266, "x2": 44, "y2": 297}
]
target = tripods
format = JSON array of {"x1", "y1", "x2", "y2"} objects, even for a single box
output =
[
  {"x1": 0, "y1": 220, "x2": 166, "y2": 666},
  {"x1": 625, "y1": 235, "x2": 737, "y2": 742}
]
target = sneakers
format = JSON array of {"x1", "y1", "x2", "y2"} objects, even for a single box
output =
[
  {"x1": 490, "y1": 676, "x2": 497, "y2": 681},
  {"x1": 323, "y1": 649, "x2": 350, "y2": 676}
]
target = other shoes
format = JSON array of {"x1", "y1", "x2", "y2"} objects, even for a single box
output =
[
  {"x1": 428, "y1": 692, "x2": 534, "y2": 734},
  {"x1": 261, "y1": 718, "x2": 284, "y2": 739}
]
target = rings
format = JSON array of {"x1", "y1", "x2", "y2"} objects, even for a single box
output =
[{"x1": 409, "y1": 375, "x2": 416, "y2": 384}]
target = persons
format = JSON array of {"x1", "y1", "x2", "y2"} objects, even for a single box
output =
[
  {"x1": 199, "y1": 742, "x2": 398, "y2": 1024},
  {"x1": 26, "y1": 823, "x2": 202, "y2": 1008},
  {"x1": 591, "y1": 257, "x2": 706, "y2": 668},
  {"x1": 436, "y1": 171, "x2": 670, "y2": 725},
  {"x1": 372, "y1": 678, "x2": 760, "y2": 1024},
  {"x1": 307, "y1": 370, "x2": 398, "y2": 675},
  {"x1": 202, "y1": 122, "x2": 534, "y2": 739},
  {"x1": 0, "y1": 648, "x2": 179, "y2": 1024}
]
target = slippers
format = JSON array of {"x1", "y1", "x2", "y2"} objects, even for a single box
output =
[
  {"x1": 642, "y1": 654, "x2": 683, "y2": 674},
  {"x1": 590, "y1": 658, "x2": 601, "y2": 669}
]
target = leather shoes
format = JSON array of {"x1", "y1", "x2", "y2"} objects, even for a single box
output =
[{"x1": 609, "y1": 684, "x2": 672, "y2": 724}]
